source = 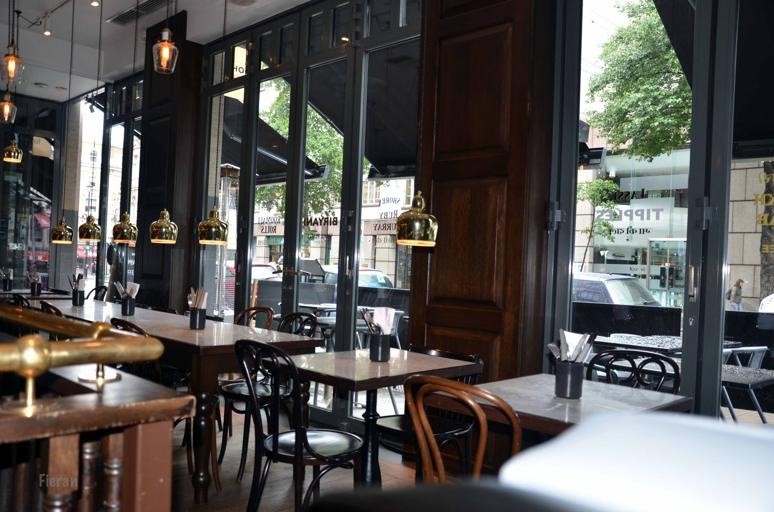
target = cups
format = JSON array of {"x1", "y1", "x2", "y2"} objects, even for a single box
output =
[
  {"x1": 187, "y1": 306, "x2": 206, "y2": 331},
  {"x1": 120, "y1": 298, "x2": 135, "y2": 316},
  {"x1": 1, "y1": 278, "x2": 12, "y2": 291},
  {"x1": 554, "y1": 359, "x2": 583, "y2": 399},
  {"x1": 28, "y1": 281, "x2": 42, "y2": 298},
  {"x1": 369, "y1": 333, "x2": 392, "y2": 363},
  {"x1": 70, "y1": 290, "x2": 85, "y2": 307}
]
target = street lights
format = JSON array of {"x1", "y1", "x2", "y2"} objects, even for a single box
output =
[
  {"x1": 85, "y1": 138, "x2": 100, "y2": 215},
  {"x1": 218, "y1": 161, "x2": 238, "y2": 316}
]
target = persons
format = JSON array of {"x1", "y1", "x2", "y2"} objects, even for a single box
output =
[{"x1": 725, "y1": 279, "x2": 745, "y2": 311}]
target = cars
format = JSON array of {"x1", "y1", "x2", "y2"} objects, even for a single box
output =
[
  {"x1": 757, "y1": 292, "x2": 774, "y2": 332},
  {"x1": 25, "y1": 215, "x2": 670, "y2": 354}
]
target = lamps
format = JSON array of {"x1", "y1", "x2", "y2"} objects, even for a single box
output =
[
  {"x1": 110, "y1": 3, "x2": 139, "y2": 245},
  {"x1": 389, "y1": 189, "x2": 437, "y2": 248},
  {"x1": 1, "y1": 91, "x2": 19, "y2": 126},
  {"x1": 146, "y1": 208, "x2": 180, "y2": 245},
  {"x1": 46, "y1": 3, "x2": 76, "y2": 245},
  {"x1": 76, "y1": 4, "x2": 106, "y2": 246},
  {"x1": 1, "y1": 1, "x2": 26, "y2": 84},
  {"x1": 193, "y1": 0, "x2": 232, "y2": 246},
  {"x1": 150, "y1": 3, "x2": 180, "y2": 73},
  {"x1": 4, "y1": 134, "x2": 24, "y2": 168}
]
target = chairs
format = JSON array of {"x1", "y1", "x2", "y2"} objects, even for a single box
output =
[{"x1": 13, "y1": 286, "x2": 767, "y2": 512}]
